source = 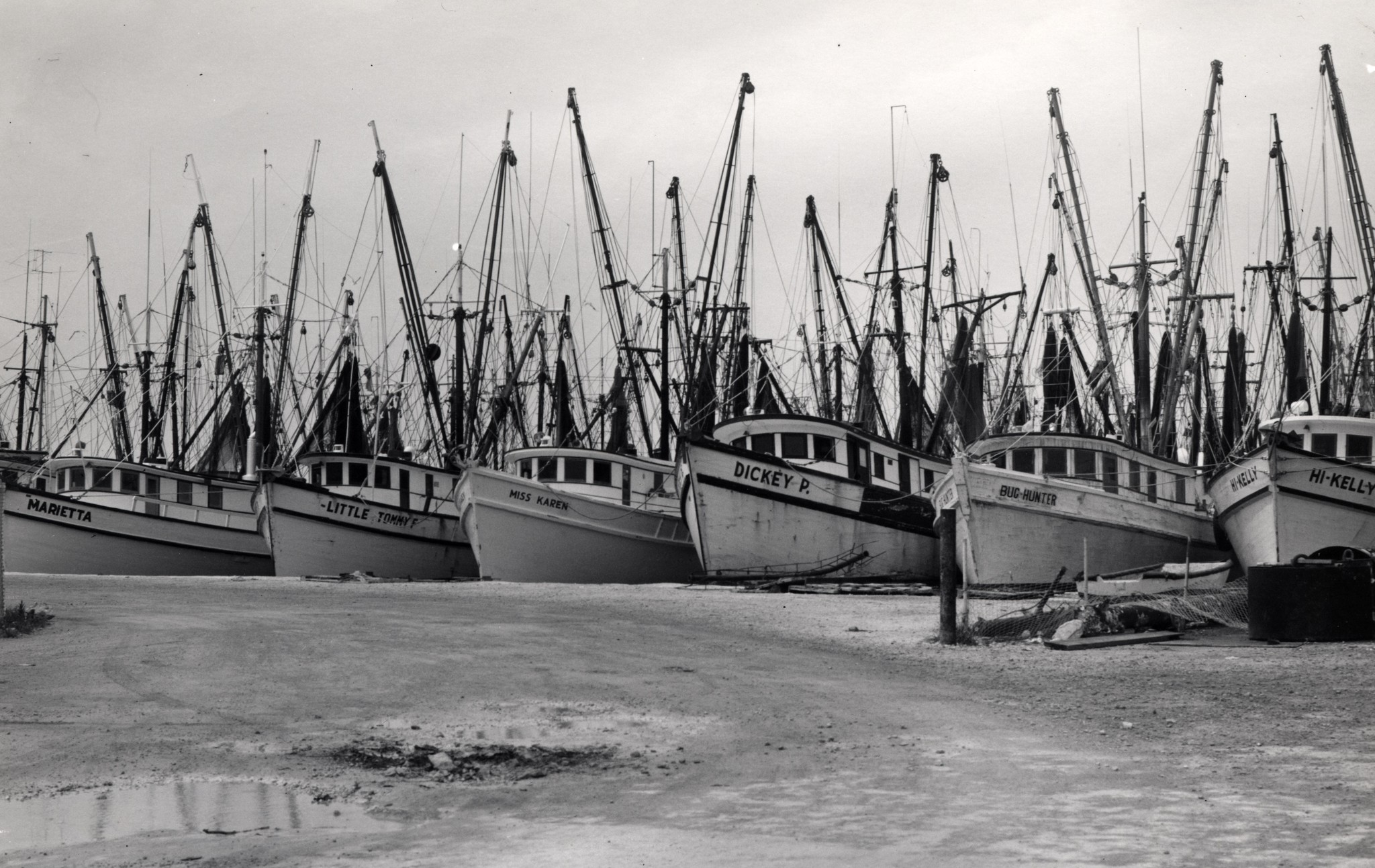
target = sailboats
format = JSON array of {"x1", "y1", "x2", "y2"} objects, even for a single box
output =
[{"x1": 0, "y1": 45, "x2": 1374, "y2": 596}]
